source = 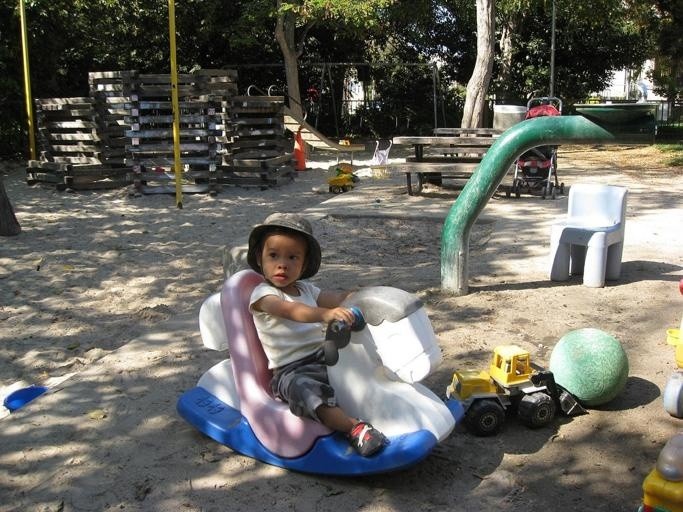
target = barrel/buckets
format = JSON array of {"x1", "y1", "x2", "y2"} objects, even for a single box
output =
[{"x1": 2, "y1": 384, "x2": 49, "y2": 414}]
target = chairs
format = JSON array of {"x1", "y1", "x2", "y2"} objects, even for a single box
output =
[{"x1": 548, "y1": 184, "x2": 628, "y2": 287}]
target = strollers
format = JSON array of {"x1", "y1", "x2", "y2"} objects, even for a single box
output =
[{"x1": 505, "y1": 97, "x2": 564, "y2": 199}]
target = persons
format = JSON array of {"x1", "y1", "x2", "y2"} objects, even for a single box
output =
[{"x1": 245, "y1": 207, "x2": 389, "y2": 457}]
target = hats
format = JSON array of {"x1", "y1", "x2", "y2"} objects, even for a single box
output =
[{"x1": 247, "y1": 213, "x2": 321, "y2": 281}]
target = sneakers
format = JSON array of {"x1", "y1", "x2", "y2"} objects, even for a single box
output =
[{"x1": 344, "y1": 417, "x2": 389, "y2": 457}]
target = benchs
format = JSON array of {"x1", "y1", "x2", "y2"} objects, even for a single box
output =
[{"x1": 387, "y1": 127, "x2": 518, "y2": 196}]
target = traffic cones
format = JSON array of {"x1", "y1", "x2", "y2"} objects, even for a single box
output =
[{"x1": 292, "y1": 129, "x2": 313, "y2": 171}]
